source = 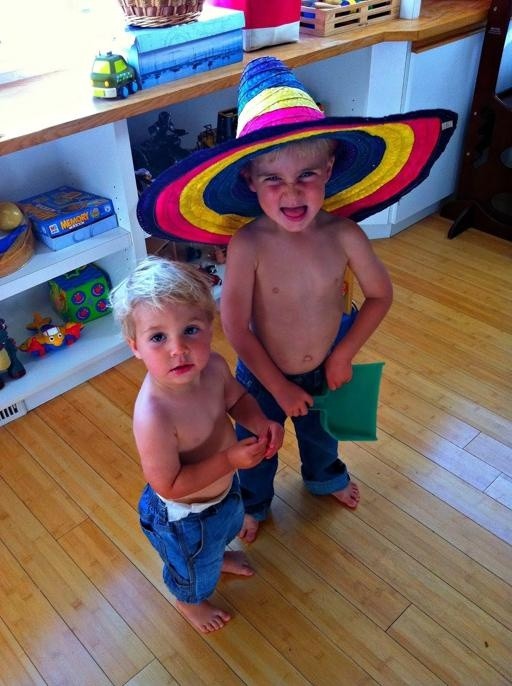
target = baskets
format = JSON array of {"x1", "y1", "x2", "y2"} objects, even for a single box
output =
[{"x1": 118, "y1": 0, "x2": 204, "y2": 27}]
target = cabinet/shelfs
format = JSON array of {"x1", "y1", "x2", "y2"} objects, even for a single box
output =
[
  {"x1": 387, "y1": 16, "x2": 487, "y2": 245},
  {"x1": 0, "y1": 113, "x2": 148, "y2": 429},
  {"x1": 124, "y1": 40, "x2": 386, "y2": 302}
]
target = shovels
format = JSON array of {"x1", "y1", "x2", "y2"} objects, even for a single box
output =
[{"x1": 308, "y1": 362, "x2": 386, "y2": 441}]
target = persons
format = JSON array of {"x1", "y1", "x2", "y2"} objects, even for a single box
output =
[
  {"x1": 106, "y1": 257, "x2": 252, "y2": 633},
  {"x1": 219, "y1": 137, "x2": 393, "y2": 547}
]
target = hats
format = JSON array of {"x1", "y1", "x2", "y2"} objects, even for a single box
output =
[{"x1": 138, "y1": 56, "x2": 458, "y2": 247}]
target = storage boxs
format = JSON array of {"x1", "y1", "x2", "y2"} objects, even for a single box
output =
[{"x1": 101, "y1": 6, "x2": 247, "y2": 89}]
